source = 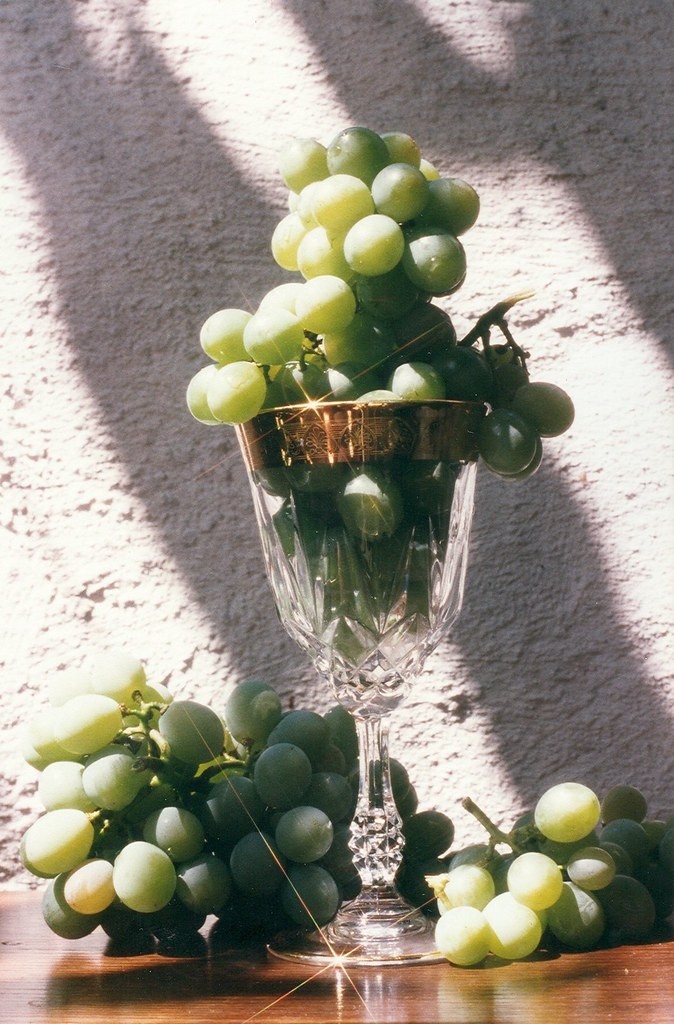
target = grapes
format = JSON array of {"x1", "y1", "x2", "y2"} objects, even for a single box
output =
[
  {"x1": 187, "y1": 127, "x2": 576, "y2": 666},
  {"x1": 16, "y1": 656, "x2": 674, "y2": 968}
]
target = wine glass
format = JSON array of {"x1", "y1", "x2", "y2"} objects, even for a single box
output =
[{"x1": 233, "y1": 399, "x2": 493, "y2": 967}]
